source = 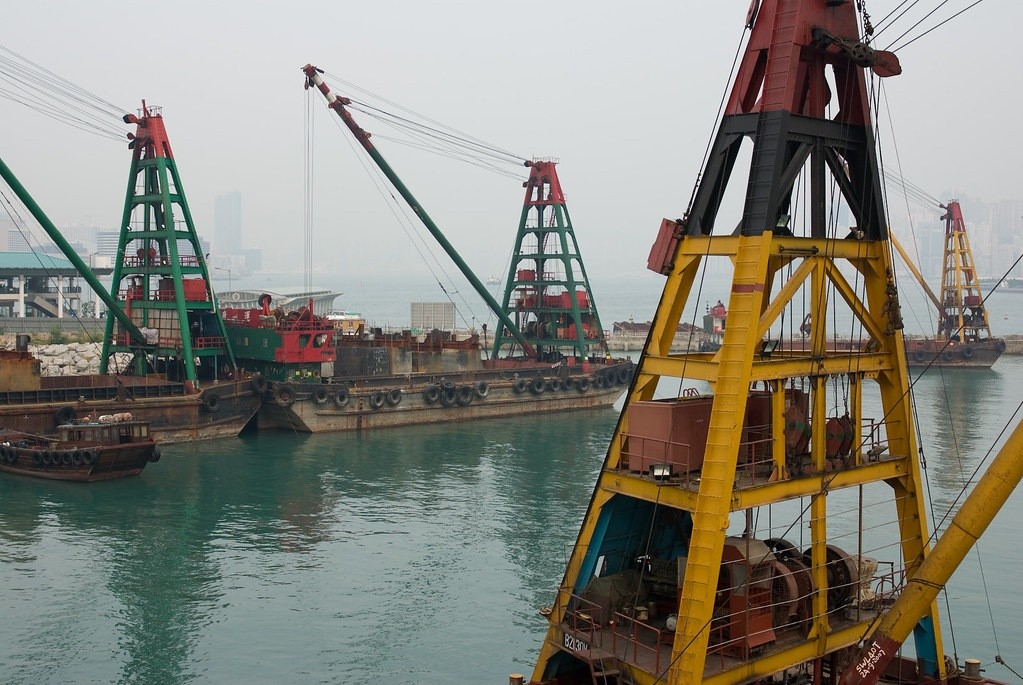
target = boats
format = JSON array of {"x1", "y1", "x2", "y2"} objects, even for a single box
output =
[
  {"x1": 0, "y1": 43, "x2": 268, "y2": 449},
  {"x1": 194, "y1": 62, "x2": 640, "y2": 434},
  {"x1": 0, "y1": 410, "x2": 162, "y2": 483},
  {"x1": 696, "y1": 142, "x2": 1008, "y2": 369}
]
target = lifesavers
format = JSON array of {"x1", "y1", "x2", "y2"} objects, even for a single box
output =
[
  {"x1": 370, "y1": 392, "x2": 385, "y2": 408},
  {"x1": 604, "y1": 369, "x2": 617, "y2": 388},
  {"x1": 474, "y1": 381, "x2": 490, "y2": 399},
  {"x1": 251, "y1": 375, "x2": 268, "y2": 394},
  {"x1": 617, "y1": 367, "x2": 628, "y2": 384},
  {"x1": 423, "y1": 385, "x2": 441, "y2": 403},
  {"x1": 433, "y1": 380, "x2": 455, "y2": 390},
  {"x1": 514, "y1": 379, "x2": 528, "y2": 392},
  {"x1": 577, "y1": 378, "x2": 590, "y2": 392},
  {"x1": 442, "y1": 389, "x2": 457, "y2": 406},
  {"x1": 203, "y1": 392, "x2": 222, "y2": 412},
  {"x1": 594, "y1": 376, "x2": 604, "y2": 388},
  {"x1": 563, "y1": 377, "x2": 574, "y2": 390},
  {"x1": 530, "y1": 377, "x2": 546, "y2": 394},
  {"x1": 313, "y1": 369, "x2": 320, "y2": 378},
  {"x1": 334, "y1": 388, "x2": 350, "y2": 408},
  {"x1": 311, "y1": 385, "x2": 329, "y2": 405},
  {"x1": 549, "y1": 379, "x2": 561, "y2": 391},
  {"x1": 274, "y1": 386, "x2": 295, "y2": 407},
  {"x1": 456, "y1": 384, "x2": 474, "y2": 406},
  {"x1": 386, "y1": 389, "x2": 402, "y2": 405}
]
